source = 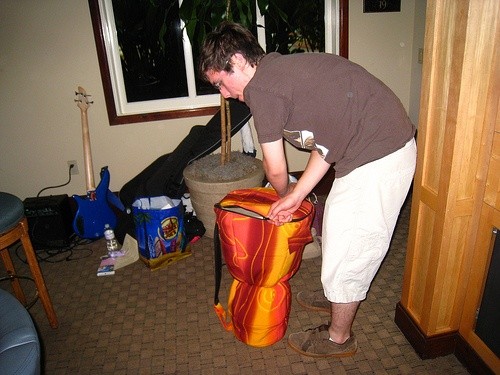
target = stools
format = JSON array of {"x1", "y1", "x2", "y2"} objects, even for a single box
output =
[
  {"x1": 0, "y1": 192, "x2": 59, "y2": 329},
  {"x1": 0, "y1": 288, "x2": 42, "y2": 375}
]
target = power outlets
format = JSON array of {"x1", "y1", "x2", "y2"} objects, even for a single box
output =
[{"x1": 68, "y1": 160, "x2": 80, "y2": 175}]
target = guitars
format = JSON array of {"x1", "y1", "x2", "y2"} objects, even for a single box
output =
[{"x1": 73, "y1": 86, "x2": 116, "y2": 240}]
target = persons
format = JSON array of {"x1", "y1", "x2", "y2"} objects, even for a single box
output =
[{"x1": 198, "y1": 21, "x2": 418, "y2": 358}]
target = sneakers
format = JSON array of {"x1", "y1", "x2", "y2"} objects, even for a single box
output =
[
  {"x1": 287, "y1": 323, "x2": 358, "y2": 358},
  {"x1": 297, "y1": 289, "x2": 332, "y2": 313}
]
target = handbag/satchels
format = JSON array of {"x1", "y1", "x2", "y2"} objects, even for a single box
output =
[{"x1": 131, "y1": 195, "x2": 193, "y2": 272}]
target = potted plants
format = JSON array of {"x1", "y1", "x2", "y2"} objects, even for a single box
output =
[{"x1": 162, "y1": 1, "x2": 290, "y2": 238}]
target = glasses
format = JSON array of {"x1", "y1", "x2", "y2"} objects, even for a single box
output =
[{"x1": 214, "y1": 56, "x2": 231, "y2": 91}]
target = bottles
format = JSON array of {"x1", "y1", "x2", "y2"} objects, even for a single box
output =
[{"x1": 104, "y1": 224, "x2": 117, "y2": 251}]
